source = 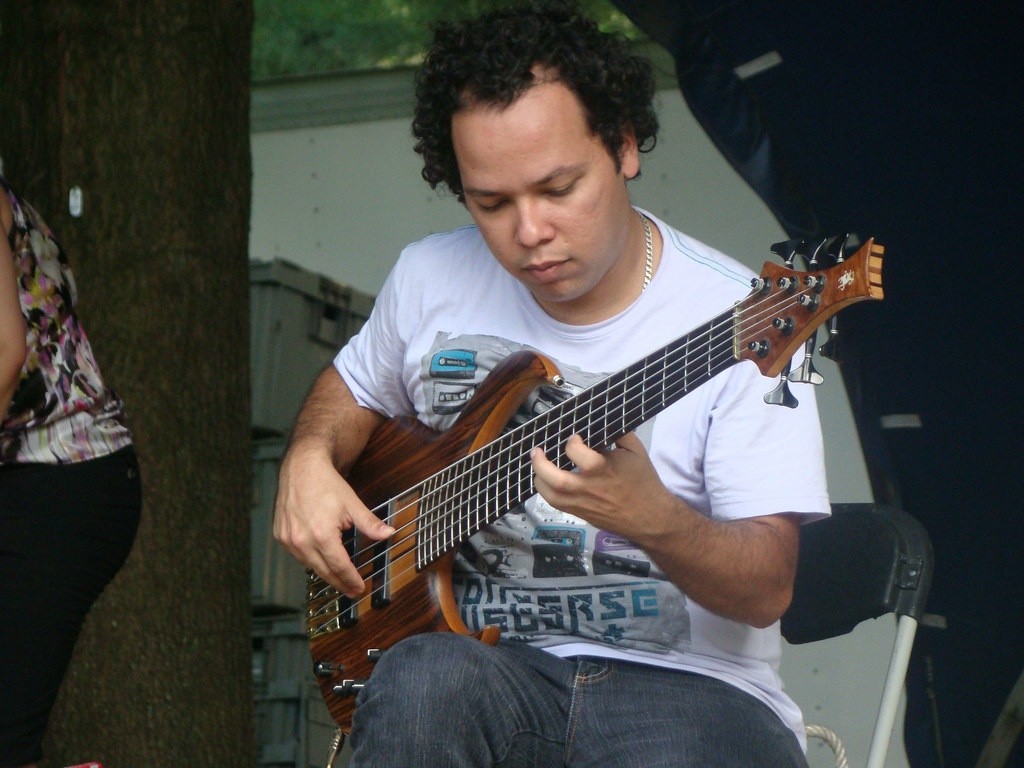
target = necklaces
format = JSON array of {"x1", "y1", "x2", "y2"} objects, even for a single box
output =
[{"x1": 632, "y1": 207, "x2": 653, "y2": 296}]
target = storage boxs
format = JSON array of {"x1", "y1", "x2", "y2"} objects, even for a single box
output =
[{"x1": 251, "y1": 258, "x2": 375, "y2": 768}]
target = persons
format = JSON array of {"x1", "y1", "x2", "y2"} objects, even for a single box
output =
[
  {"x1": 0, "y1": 175, "x2": 144, "y2": 768},
  {"x1": 270, "y1": 1, "x2": 833, "y2": 768}
]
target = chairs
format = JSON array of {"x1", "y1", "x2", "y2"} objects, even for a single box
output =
[{"x1": 782, "y1": 501, "x2": 936, "y2": 768}]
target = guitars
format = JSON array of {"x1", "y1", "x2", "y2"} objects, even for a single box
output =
[{"x1": 304, "y1": 233, "x2": 886, "y2": 729}]
